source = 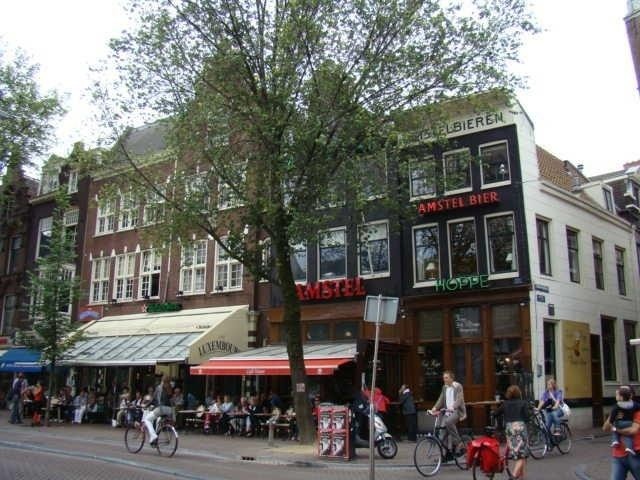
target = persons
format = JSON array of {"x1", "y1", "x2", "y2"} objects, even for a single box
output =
[
  {"x1": 7, "y1": 371, "x2": 319, "y2": 445},
  {"x1": 427, "y1": 370, "x2": 468, "y2": 463},
  {"x1": 596, "y1": 384, "x2": 640, "y2": 480},
  {"x1": 355, "y1": 383, "x2": 419, "y2": 443},
  {"x1": 536, "y1": 378, "x2": 567, "y2": 452},
  {"x1": 489, "y1": 385, "x2": 530, "y2": 480}
]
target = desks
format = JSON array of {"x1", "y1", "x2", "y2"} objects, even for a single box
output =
[{"x1": 179, "y1": 409, "x2": 294, "y2": 440}]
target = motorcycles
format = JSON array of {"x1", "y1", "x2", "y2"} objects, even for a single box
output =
[{"x1": 349, "y1": 404, "x2": 398, "y2": 459}]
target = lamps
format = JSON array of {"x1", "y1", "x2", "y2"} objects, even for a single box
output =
[{"x1": 426, "y1": 262, "x2": 439, "y2": 281}]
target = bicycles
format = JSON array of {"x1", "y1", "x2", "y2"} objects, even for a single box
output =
[
  {"x1": 124, "y1": 405, "x2": 179, "y2": 458},
  {"x1": 413, "y1": 407, "x2": 572, "y2": 480}
]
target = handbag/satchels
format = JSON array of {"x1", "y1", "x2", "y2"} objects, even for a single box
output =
[{"x1": 562, "y1": 404, "x2": 571, "y2": 416}]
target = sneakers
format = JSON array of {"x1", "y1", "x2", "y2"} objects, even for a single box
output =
[
  {"x1": 610, "y1": 440, "x2": 636, "y2": 458},
  {"x1": 551, "y1": 431, "x2": 562, "y2": 437},
  {"x1": 150, "y1": 435, "x2": 158, "y2": 445},
  {"x1": 456, "y1": 441, "x2": 465, "y2": 449}
]
